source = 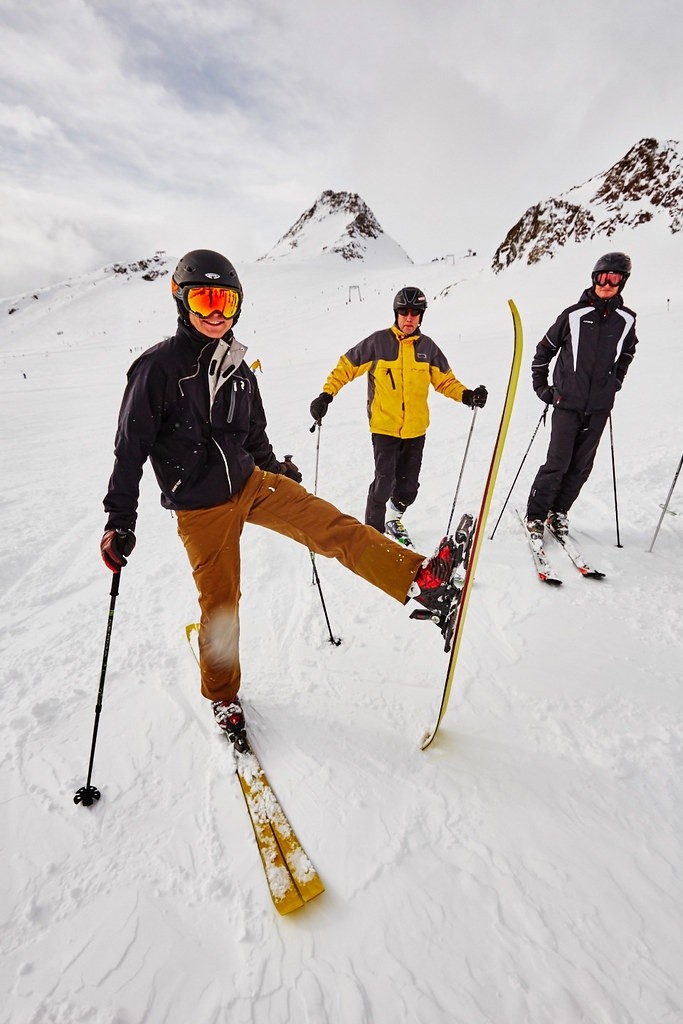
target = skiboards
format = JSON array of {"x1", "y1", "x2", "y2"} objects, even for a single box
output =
[
  {"x1": 182, "y1": 297, "x2": 524, "y2": 917},
  {"x1": 512, "y1": 503, "x2": 606, "y2": 586}
]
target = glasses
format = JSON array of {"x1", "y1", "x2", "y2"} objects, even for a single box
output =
[
  {"x1": 396, "y1": 308, "x2": 422, "y2": 316},
  {"x1": 594, "y1": 271, "x2": 627, "y2": 287},
  {"x1": 183, "y1": 285, "x2": 243, "y2": 320}
]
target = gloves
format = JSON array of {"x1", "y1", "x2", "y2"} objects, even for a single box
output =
[
  {"x1": 310, "y1": 392, "x2": 333, "y2": 427},
  {"x1": 462, "y1": 388, "x2": 488, "y2": 408},
  {"x1": 266, "y1": 453, "x2": 302, "y2": 484},
  {"x1": 537, "y1": 385, "x2": 554, "y2": 404},
  {"x1": 100, "y1": 526, "x2": 136, "y2": 574}
]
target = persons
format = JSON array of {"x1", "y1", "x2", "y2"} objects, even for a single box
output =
[
  {"x1": 310, "y1": 287, "x2": 488, "y2": 547},
  {"x1": 101, "y1": 248, "x2": 466, "y2": 735},
  {"x1": 523, "y1": 253, "x2": 640, "y2": 545}
]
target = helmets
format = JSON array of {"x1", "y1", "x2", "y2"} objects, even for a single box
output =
[
  {"x1": 171, "y1": 249, "x2": 244, "y2": 327},
  {"x1": 393, "y1": 287, "x2": 426, "y2": 311},
  {"x1": 592, "y1": 253, "x2": 631, "y2": 280}
]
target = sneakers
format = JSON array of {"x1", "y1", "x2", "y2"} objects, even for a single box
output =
[
  {"x1": 212, "y1": 694, "x2": 246, "y2": 731},
  {"x1": 386, "y1": 506, "x2": 413, "y2": 546},
  {"x1": 407, "y1": 513, "x2": 473, "y2": 619},
  {"x1": 526, "y1": 519, "x2": 544, "y2": 536},
  {"x1": 546, "y1": 508, "x2": 570, "y2": 536}
]
